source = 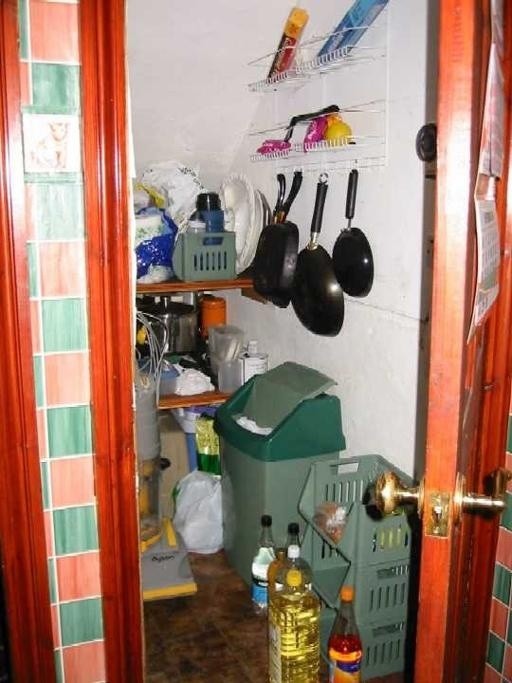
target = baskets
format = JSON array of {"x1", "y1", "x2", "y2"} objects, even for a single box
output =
[
  {"x1": 171, "y1": 231, "x2": 237, "y2": 280},
  {"x1": 318, "y1": 608, "x2": 405, "y2": 682},
  {"x1": 298, "y1": 526, "x2": 408, "y2": 621},
  {"x1": 295, "y1": 451, "x2": 418, "y2": 566}
]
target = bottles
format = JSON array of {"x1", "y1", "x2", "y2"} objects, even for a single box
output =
[
  {"x1": 328, "y1": 587, "x2": 362, "y2": 682},
  {"x1": 250, "y1": 515, "x2": 319, "y2": 683},
  {"x1": 195, "y1": 192, "x2": 225, "y2": 270},
  {"x1": 201, "y1": 295, "x2": 227, "y2": 352},
  {"x1": 239, "y1": 340, "x2": 268, "y2": 386}
]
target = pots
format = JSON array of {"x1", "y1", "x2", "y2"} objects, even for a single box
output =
[
  {"x1": 251, "y1": 169, "x2": 374, "y2": 337},
  {"x1": 149, "y1": 303, "x2": 198, "y2": 356}
]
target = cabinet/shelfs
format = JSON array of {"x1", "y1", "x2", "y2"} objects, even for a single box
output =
[
  {"x1": 296, "y1": 454, "x2": 420, "y2": 683},
  {"x1": 138, "y1": 277, "x2": 265, "y2": 411},
  {"x1": 246, "y1": 2, "x2": 389, "y2": 176}
]
